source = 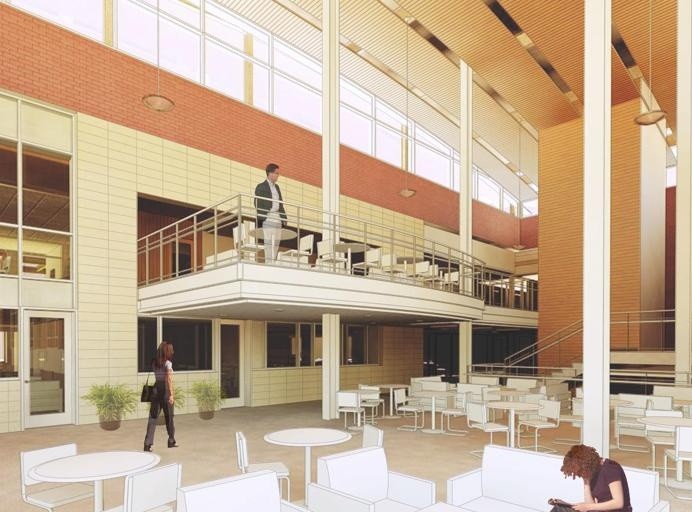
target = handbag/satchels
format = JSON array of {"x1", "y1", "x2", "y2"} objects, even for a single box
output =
[{"x1": 141, "y1": 385, "x2": 153, "y2": 402}]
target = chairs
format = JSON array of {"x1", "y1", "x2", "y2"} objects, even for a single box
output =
[
  {"x1": 19, "y1": 371, "x2": 692, "y2": 510},
  {"x1": 202, "y1": 218, "x2": 479, "y2": 295}
]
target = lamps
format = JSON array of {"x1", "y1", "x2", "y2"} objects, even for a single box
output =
[
  {"x1": 141, "y1": 1, "x2": 176, "y2": 116},
  {"x1": 631, "y1": 0, "x2": 671, "y2": 126}
]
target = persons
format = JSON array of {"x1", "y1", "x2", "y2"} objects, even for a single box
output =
[
  {"x1": 547, "y1": 444, "x2": 632, "y2": 512},
  {"x1": 254, "y1": 163, "x2": 287, "y2": 263},
  {"x1": 144, "y1": 340, "x2": 179, "y2": 451}
]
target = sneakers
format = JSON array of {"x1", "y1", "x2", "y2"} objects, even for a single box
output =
[
  {"x1": 168, "y1": 441, "x2": 179, "y2": 448},
  {"x1": 144, "y1": 445, "x2": 153, "y2": 452}
]
